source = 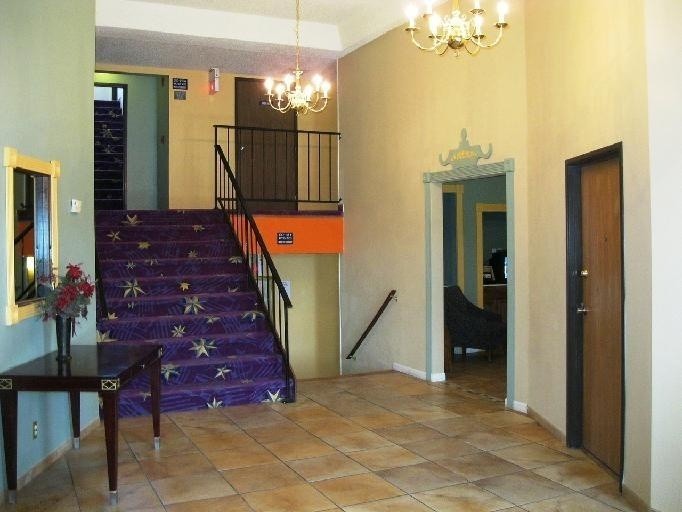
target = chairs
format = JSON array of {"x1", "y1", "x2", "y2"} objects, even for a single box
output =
[{"x1": 445, "y1": 285, "x2": 503, "y2": 364}]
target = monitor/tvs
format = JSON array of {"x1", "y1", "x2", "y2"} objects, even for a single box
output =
[{"x1": 488, "y1": 250, "x2": 507, "y2": 284}]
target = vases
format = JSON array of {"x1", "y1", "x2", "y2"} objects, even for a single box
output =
[{"x1": 56, "y1": 314, "x2": 73, "y2": 361}]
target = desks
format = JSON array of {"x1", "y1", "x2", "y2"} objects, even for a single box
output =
[{"x1": 2, "y1": 343, "x2": 167, "y2": 508}]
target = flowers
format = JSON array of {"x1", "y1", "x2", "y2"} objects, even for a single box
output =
[{"x1": 39, "y1": 263, "x2": 96, "y2": 338}]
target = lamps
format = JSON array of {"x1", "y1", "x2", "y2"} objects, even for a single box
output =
[
  {"x1": 404, "y1": 0, "x2": 508, "y2": 57},
  {"x1": 263, "y1": 1, "x2": 332, "y2": 116}
]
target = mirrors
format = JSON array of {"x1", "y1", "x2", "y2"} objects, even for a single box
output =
[{"x1": 4, "y1": 146, "x2": 60, "y2": 327}]
